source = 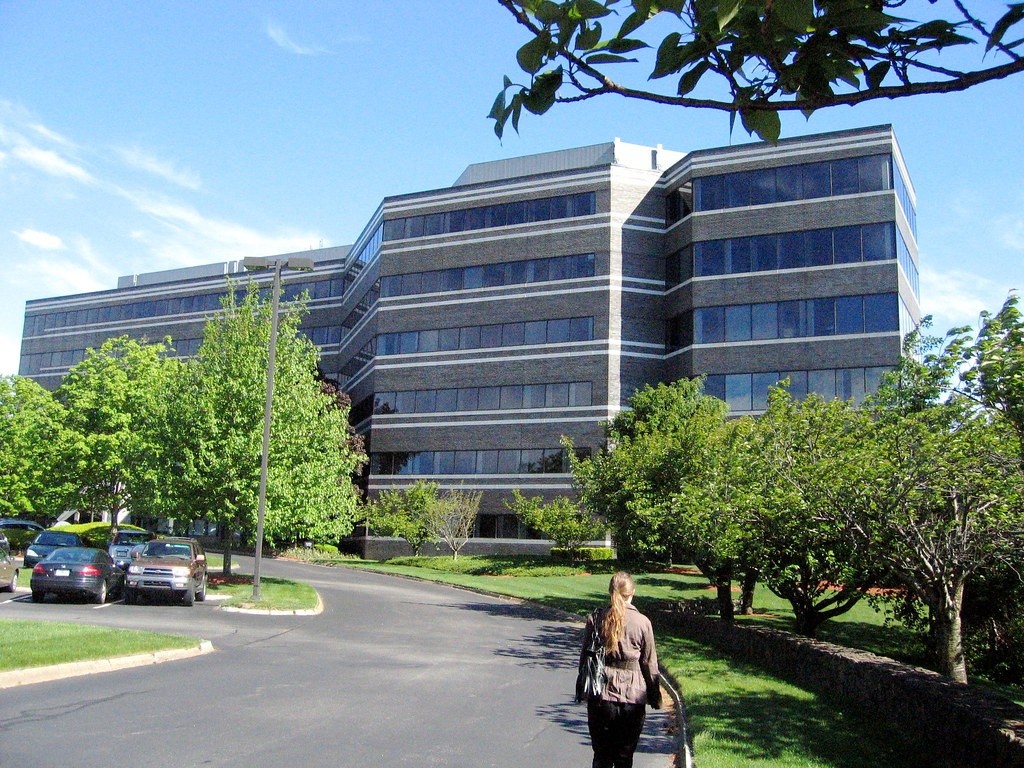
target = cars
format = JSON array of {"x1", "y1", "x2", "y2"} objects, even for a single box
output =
[
  {"x1": 29, "y1": 546, "x2": 128, "y2": 605},
  {"x1": 107, "y1": 530, "x2": 156, "y2": 570},
  {"x1": 0, "y1": 547, "x2": 19, "y2": 593},
  {"x1": 23, "y1": 530, "x2": 86, "y2": 569}
]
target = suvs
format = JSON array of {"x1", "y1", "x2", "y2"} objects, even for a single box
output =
[{"x1": 123, "y1": 536, "x2": 209, "y2": 607}]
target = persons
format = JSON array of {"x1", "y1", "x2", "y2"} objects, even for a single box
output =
[{"x1": 579, "y1": 571, "x2": 664, "y2": 768}]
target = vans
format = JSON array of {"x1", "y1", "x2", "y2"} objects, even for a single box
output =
[{"x1": 0, "y1": 517, "x2": 45, "y2": 532}]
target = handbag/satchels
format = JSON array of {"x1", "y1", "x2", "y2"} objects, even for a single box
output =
[{"x1": 575, "y1": 612, "x2": 605, "y2": 704}]
